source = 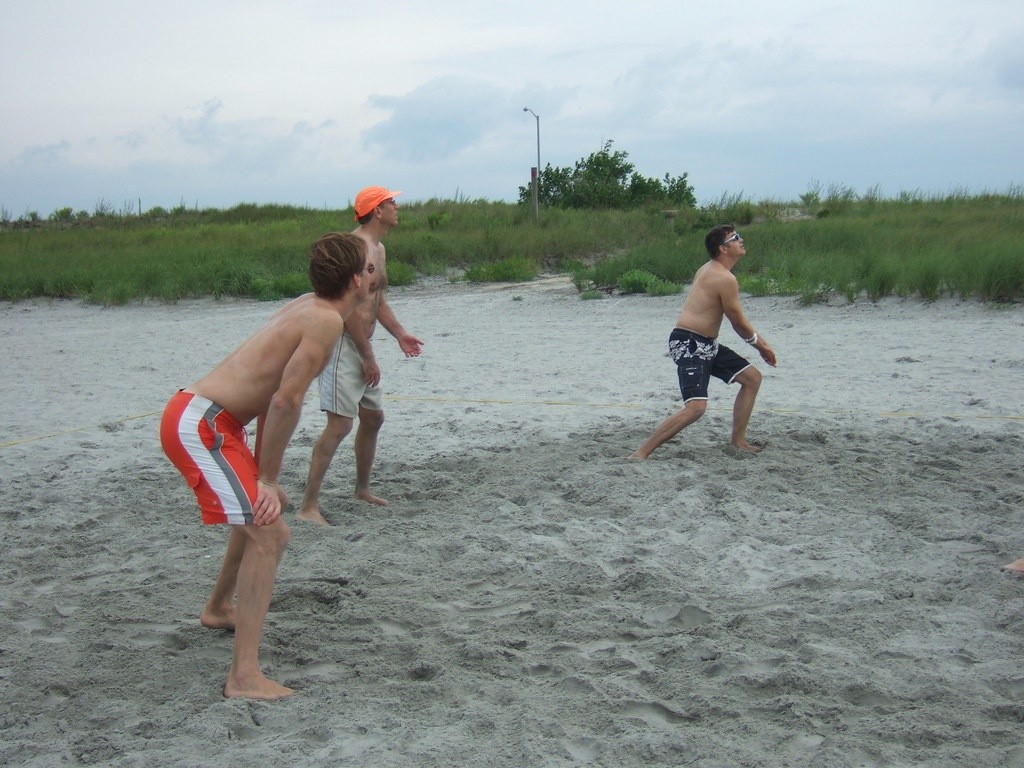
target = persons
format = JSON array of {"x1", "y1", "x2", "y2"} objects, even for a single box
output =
[
  {"x1": 630, "y1": 224, "x2": 777, "y2": 459},
  {"x1": 296, "y1": 186, "x2": 425, "y2": 524},
  {"x1": 160, "y1": 233, "x2": 373, "y2": 700}
]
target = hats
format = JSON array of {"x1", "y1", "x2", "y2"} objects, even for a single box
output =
[{"x1": 353, "y1": 186, "x2": 400, "y2": 221}]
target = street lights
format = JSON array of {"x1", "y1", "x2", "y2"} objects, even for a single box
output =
[{"x1": 523, "y1": 107, "x2": 540, "y2": 200}]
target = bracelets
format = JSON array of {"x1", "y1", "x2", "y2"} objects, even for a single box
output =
[{"x1": 746, "y1": 333, "x2": 757, "y2": 345}]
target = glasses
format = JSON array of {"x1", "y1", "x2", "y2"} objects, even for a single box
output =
[{"x1": 723, "y1": 234, "x2": 739, "y2": 244}]
